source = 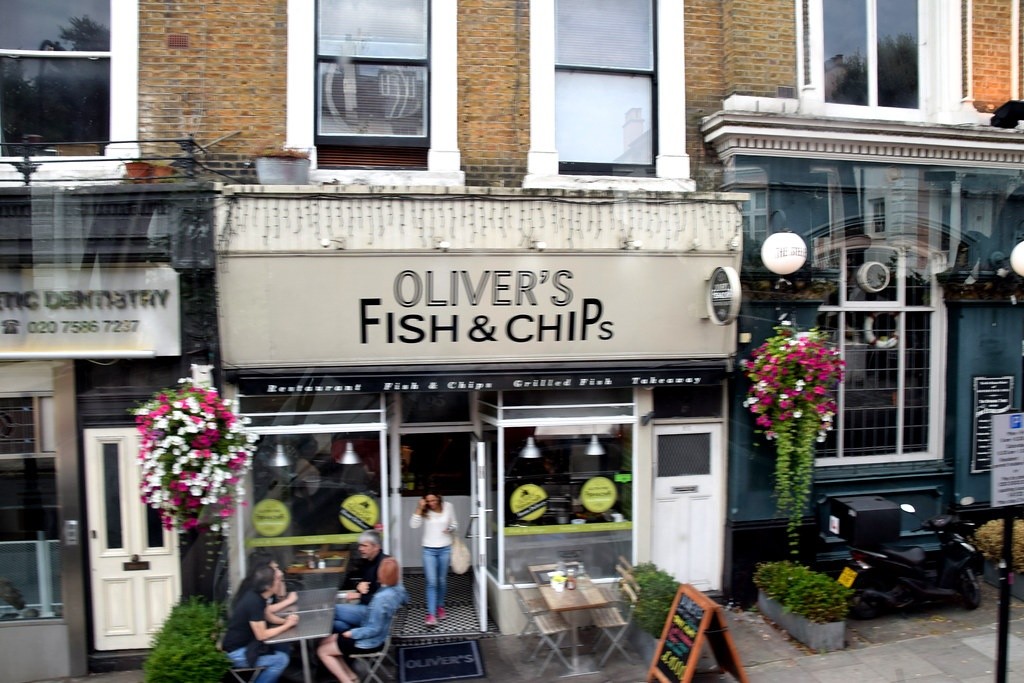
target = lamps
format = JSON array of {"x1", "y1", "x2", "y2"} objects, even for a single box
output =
[
  {"x1": 268, "y1": 444, "x2": 292, "y2": 467},
  {"x1": 584, "y1": 435, "x2": 605, "y2": 456},
  {"x1": 989, "y1": 234, "x2": 1024, "y2": 277},
  {"x1": 761, "y1": 210, "x2": 807, "y2": 274},
  {"x1": 334, "y1": 442, "x2": 362, "y2": 464},
  {"x1": 518, "y1": 437, "x2": 541, "y2": 458}
]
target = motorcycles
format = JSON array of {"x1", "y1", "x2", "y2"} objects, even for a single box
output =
[{"x1": 830, "y1": 495, "x2": 980, "y2": 620}]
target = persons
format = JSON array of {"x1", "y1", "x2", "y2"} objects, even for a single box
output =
[
  {"x1": 221, "y1": 551, "x2": 300, "y2": 683},
  {"x1": 408, "y1": 486, "x2": 458, "y2": 627},
  {"x1": 315, "y1": 529, "x2": 408, "y2": 683}
]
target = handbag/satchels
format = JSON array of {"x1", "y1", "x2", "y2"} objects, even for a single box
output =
[{"x1": 450, "y1": 520, "x2": 470, "y2": 575}]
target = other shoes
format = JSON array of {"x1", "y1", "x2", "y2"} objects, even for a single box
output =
[{"x1": 352, "y1": 677, "x2": 361, "y2": 683}]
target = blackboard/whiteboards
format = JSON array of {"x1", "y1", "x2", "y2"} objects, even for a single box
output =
[{"x1": 651, "y1": 583, "x2": 749, "y2": 683}]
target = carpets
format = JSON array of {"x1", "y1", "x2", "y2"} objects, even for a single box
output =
[{"x1": 400, "y1": 640, "x2": 486, "y2": 683}]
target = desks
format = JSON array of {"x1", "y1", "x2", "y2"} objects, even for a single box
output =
[
  {"x1": 264, "y1": 587, "x2": 338, "y2": 683},
  {"x1": 528, "y1": 562, "x2": 609, "y2": 678}
]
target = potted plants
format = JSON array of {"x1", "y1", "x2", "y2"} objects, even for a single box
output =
[
  {"x1": 243, "y1": 146, "x2": 311, "y2": 186},
  {"x1": 116, "y1": 155, "x2": 185, "y2": 184},
  {"x1": 966, "y1": 519, "x2": 1024, "y2": 601},
  {"x1": 617, "y1": 559, "x2": 683, "y2": 667},
  {"x1": 753, "y1": 561, "x2": 855, "y2": 650}
]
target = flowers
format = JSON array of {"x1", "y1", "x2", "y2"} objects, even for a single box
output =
[
  {"x1": 739, "y1": 324, "x2": 847, "y2": 553},
  {"x1": 127, "y1": 376, "x2": 260, "y2": 536}
]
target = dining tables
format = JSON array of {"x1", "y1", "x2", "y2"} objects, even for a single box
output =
[{"x1": 286, "y1": 551, "x2": 349, "y2": 573}]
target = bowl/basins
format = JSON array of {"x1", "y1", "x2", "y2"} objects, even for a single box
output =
[{"x1": 324, "y1": 558, "x2": 347, "y2": 567}]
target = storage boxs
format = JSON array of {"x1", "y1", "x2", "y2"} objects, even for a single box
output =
[{"x1": 830, "y1": 497, "x2": 902, "y2": 548}]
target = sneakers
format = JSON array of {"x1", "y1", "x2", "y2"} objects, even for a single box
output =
[
  {"x1": 425, "y1": 614, "x2": 439, "y2": 627},
  {"x1": 437, "y1": 607, "x2": 445, "y2": 619}
]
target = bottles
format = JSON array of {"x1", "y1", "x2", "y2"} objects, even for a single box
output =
[
  {"x1": 566, "y1": 565, "x2": 591, "y2": 591},
  {"x1": 307, "y1": 559, "x2": 326, "y2": 569}
]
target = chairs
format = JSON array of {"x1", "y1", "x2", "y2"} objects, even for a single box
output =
[
  {"x1": 510, "y1": 565, "x2": 641, "y2": 677},
  {"x1": 349, "y1": 615, "x2": 395, "y2": 683},
  {"x1": 211, "y1": 619, "x2": 266, "y2": 683}
]
